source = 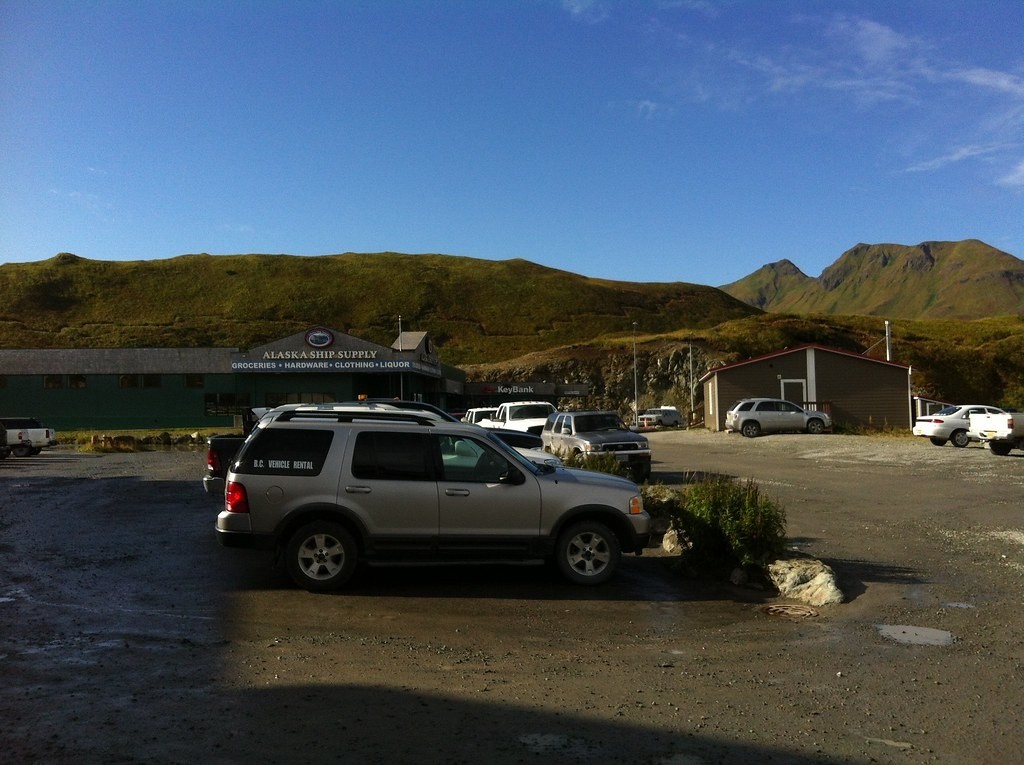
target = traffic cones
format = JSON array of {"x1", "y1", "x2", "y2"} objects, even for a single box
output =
[{"x1": 644, "y1": 417, "x2": 649, "y2": 428}]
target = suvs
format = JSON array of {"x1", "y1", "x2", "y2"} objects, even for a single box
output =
[
  {"x1": 211, "y1": 398, "x2": 654, "y2": 595},
  {"x1": 725, "y1": 397, "x2": 833, "y2": 438},
  {"x1": 539, "y1": 408, "x2": 652, "y2": 472},
  {"x1": 638, "y1": 405, "x2": 682, "y2": 428}
]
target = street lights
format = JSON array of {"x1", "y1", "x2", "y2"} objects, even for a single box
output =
[
  {"x1": 632, "y1": 320, "x2": 640, "y2": 429},
  {"x1": 689, "y1": 331, "x2": 696, "y2": 412}
]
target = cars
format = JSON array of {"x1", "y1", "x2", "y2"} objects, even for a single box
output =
[
  {"x1": 0, "y1": 421, "x2": 11, "y2": 461},
  {"x1": 0, "y1": 416, "x2": 57, "y2": 457},
  {"x1": 482, "y1": 400, "x2": 558, "y2": 438},
  {"x1": 912, "y1": 405, "x2": 1008, "y2": 448},
  {"x1": 203, "y1": 398, "x2": 564, "y2": 487},
  {"x1": 460, "y1": 407, "x2": 498, "y2": 425}
]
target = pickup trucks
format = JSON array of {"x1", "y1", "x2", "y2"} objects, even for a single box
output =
[{"x1": 967, "y1": 411, "x2": 1024, "y2": 457}]
